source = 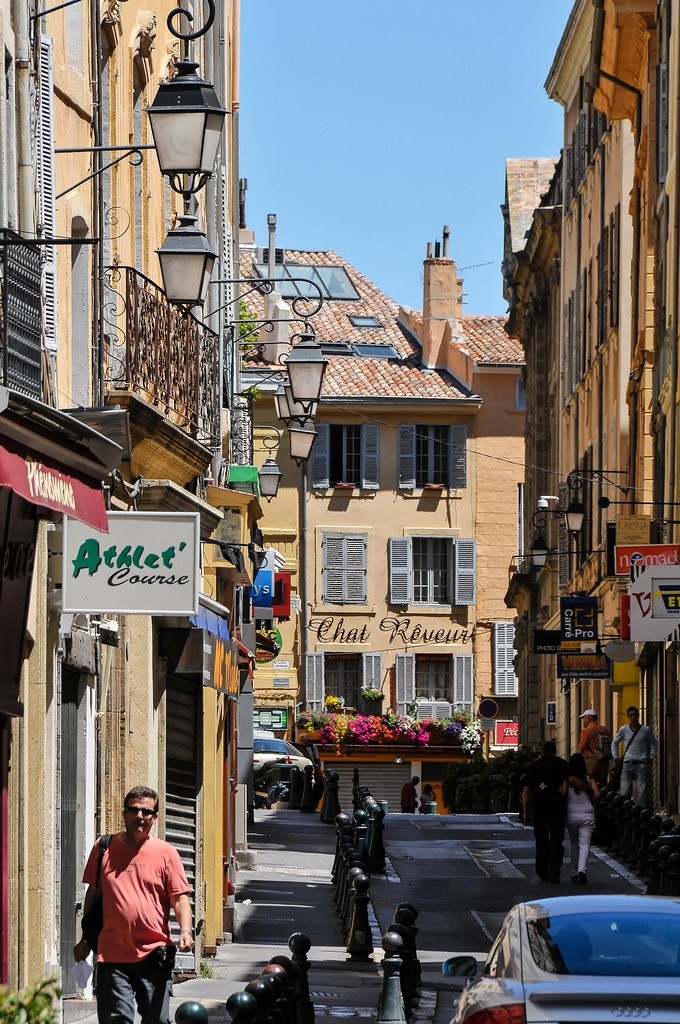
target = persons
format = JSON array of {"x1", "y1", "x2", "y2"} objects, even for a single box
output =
[
  {"x1": 561, "y1": 753, "x2": 600, "y2": 885},
  {"x1": 74, "y1": 786, "x2": 195, "y2": 1024},
  {"x1": 523, "y1": 741, "x2": 594, "y2": 885},
  {"x1": 401, "y1": 776, "x2": 419, "y2": 813},
  {"x1": 576, "y1": 710, "x2": 612, "y2": 781},
  {"x1": 612, "y1": 706, "x2": 658, "y2": 808},
  {"x1": 419, "y1": 784, "x2": 436, "y2": 814}
]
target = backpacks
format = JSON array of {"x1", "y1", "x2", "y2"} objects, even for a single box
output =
[{"x1": 585, "y1": 725, "x2": 613, "y2": 760}]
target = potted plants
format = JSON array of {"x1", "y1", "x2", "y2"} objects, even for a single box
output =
[{"x1": 362, "y1": 689, "x2": 385, "y2": 703}]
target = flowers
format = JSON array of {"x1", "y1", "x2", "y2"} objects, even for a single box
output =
[{"x1": 296, "y1": 695, "x2": 484, "y2": 754}]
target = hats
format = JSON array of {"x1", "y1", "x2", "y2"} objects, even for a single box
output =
[{"x1": 579, "y1": 709, "x2": 598, "y2": 717}]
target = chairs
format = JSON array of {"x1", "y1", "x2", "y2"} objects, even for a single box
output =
[{"x1": 555, "y1": 924, "x2": 592, "y2": 963}]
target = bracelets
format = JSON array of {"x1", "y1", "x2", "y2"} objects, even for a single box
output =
[
  {"x1": 81, "y1": 939, "x2": 87, "y2": 943},
  {"x1": 181, "y1": 931, "x2": 192, "y2": 936}
]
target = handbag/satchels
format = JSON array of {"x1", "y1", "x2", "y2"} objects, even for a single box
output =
[{"x1": 81, "y1": 833, "x2": 113, "y2": 955}]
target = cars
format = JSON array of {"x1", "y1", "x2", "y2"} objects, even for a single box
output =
[
  {"x1": 250, "y1": 738, "x2": 313, "y2": 801},
  {"x1": 441, "y1": 894, "x2": 680, "y2": 1024}
]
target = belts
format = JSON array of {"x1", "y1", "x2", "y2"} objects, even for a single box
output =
[{"x1": 624, "y1": 761, "x2": 645, "y2": 765}]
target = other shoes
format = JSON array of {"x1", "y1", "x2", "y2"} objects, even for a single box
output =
[{"x1": 571, "y1": 872, "x2": 588, "y2": 884}]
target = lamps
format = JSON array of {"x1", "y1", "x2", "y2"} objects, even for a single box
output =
[
  {"x1": 200, "y1": 536, "x2": 259, "y2": 574},
  {"x1": 395, "y1": 756, "x2": 403, "y2": 764},
  {"x1": 564, "y1": 469, "x2": 628, "y2": 539},
  {"x1": 90, "y1": 619, "x2": 119, "y2": 647},
  {"x1": 56, "y1": 145, "x2": 220, "y2": 320},
  {"x1": 529, "y1": 509, "x2": 567, "y2": 572},
  {"x1": 29, "y1": 0, "x2": 232, "y2": 209},
  {"x1": 202, "y1": 278, "x2": 329, "y2": 503}
]
